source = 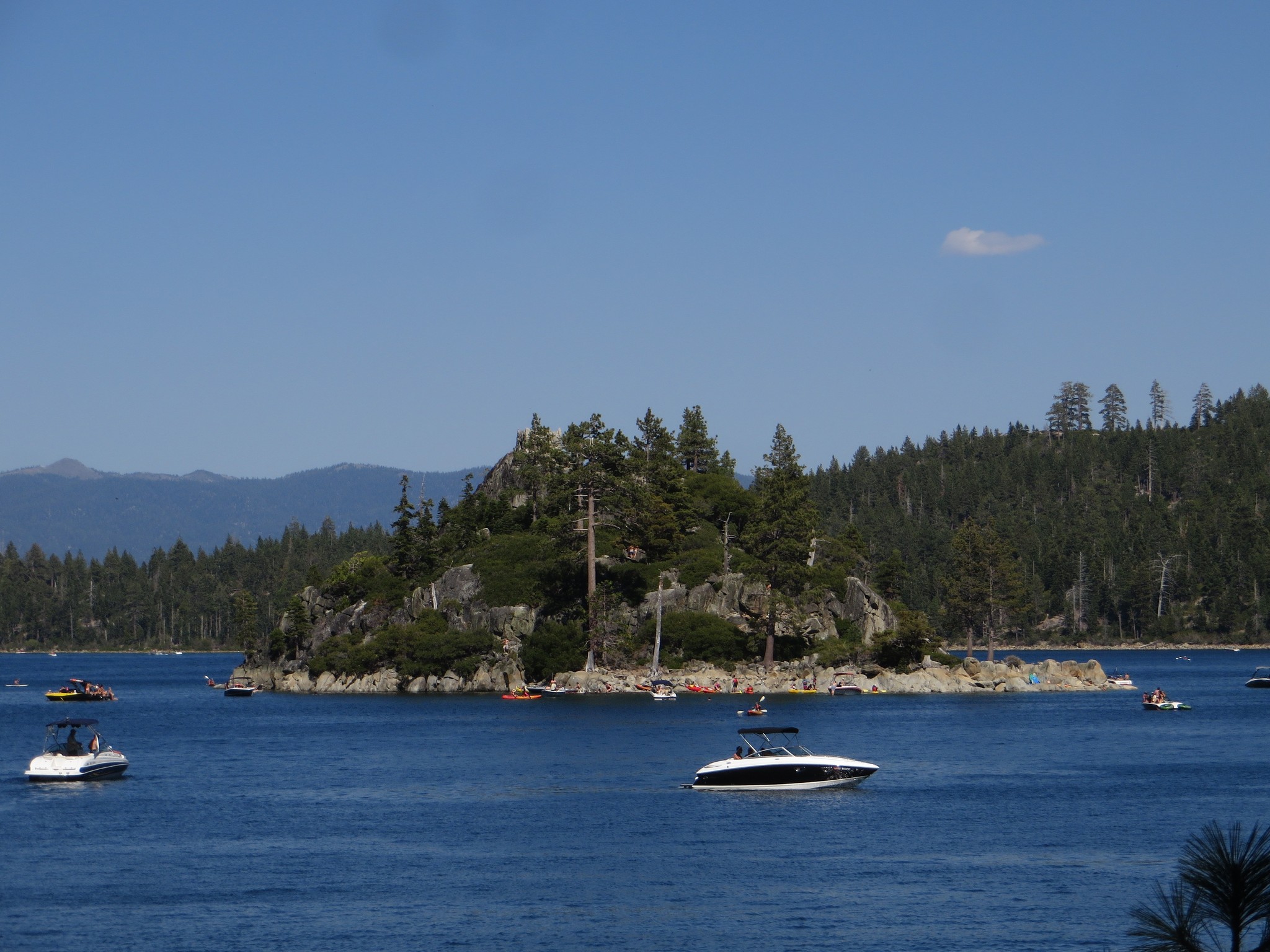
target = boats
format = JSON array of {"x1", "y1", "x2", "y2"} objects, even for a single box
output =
[
  {"x1": 692, "y1": 727, "x2": 880, "y2": 791},
  {"x1": 636, "y1": 680, "x2": 676, "y2": 700},
  {"x1": 502, "y1": 673, "x2": 586, "y2": 700},
  {"x1": 224, "y1": 677, "x2": 264, "y2": 697},
  {"x1": 828, "y1": 672, "x2": 887, "y2": 696},
  {"x1": 1029, "y1": 673, "x2": 1040, "y2": 684},
  {"x1": 208, "y1": 683, "x2": 215, "y2": 686},
  {"x1": 789, "y1": 689, "x2": 816, "y2": 695},
  {"x1": 1244, "y1": 667, "x2": 1270, "y2": 688},
  {"x1": 44, "y1": 680, "x2": 114, "y2": 702},
  {"x1": 1107, "y1": 666, "x2": 1132, "y2": 686},
  {"x1": 48, "y1": 653, "x2": 57, "y2": 657},
  {"x1": 27, "y1": 717, "x2": 129, "y2": 782},
  {"x1": 175, "y1": 651, "x2": 182, "y2": 654},
  {"x1": 1176, "y1": 658, "x2": 1190, "y2": 661},
  {"x1": 150, "y1": 649, "x2": 170, "y2": 655},
  {"x1": 1141, "y1": 698, "x2": 1192, "y2": 711},
  {"x1": 5, "y1": 685, "x2": 29, "y2": 686},
  {"x1": 747, "y1": 710, "x2": 763, "y2": 716},
  {"x1": 1225, "y1": 648, "x2": 1240, "y2": 652},
  {"x1": 685, "y1": 684, "x2": 754, "y2": 695}
]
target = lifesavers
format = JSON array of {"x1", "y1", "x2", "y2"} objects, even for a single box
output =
[
  {"x1": 1159, "y1": 704, "x2": 1174, "y2": 710},
  {"x1": 1177, "y1": 704, "x2": 1192, "y2": 709}
]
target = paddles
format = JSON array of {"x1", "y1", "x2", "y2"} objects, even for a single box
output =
[
  {"x1": 813, "y1": 688, "x2": 819, "y2": 690},
  {"x1": 752, "y1": 696, "x2": 765, "y2": 709},
  {"x1": 1176, "y1": 658, "x2": 1179, "y2": 660},
  {"x1": 708, "y1": 688, "x2": 722, "y2": 692},
  {"x1": 1031, "y1": 673, "x2": 1035, "y2": 685},
  {"x1": 1179, "y1": 657, "x2": 1190, "y2": 660},
  {"x1": 205, "y1": 676, "x2": 218, "y2": 685},
  {"x1": 737, "y1": 684, "x2": 742, "y2": 694},
  {"x1": 864, "y1": 689, "x2": 886, "y2": 692},
  {"x1": 737, "y1": 709, "x2": 767, "y2": 714}
]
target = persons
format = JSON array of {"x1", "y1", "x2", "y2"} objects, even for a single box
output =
[
  {"x1": 15, "y1": 679, "x2": 20, "y2": 685},
  {"x1": 67, "y1": 729, "x2": 82, "y2": 756},
  {"x1": 61, "y1": 681, "x2": 118, "y2": 700},
  {"x1": 501, "y1": 638, "x2": 509, "y2": 653},
  {"x1": 630, "y1": 544, "x2": 640, "y2": 559},
  {"x1": 51, "y1": 651, "x2": 55, "y2": 655},
  {"x1": 733, "y1": 746, "x2": 776, "y2": 760},
  {"x1": 1184, "y1": 655, "x2": 1186, "y2": 659},
  {"x1": 1047, "y1": 671, "x2": 1166, "y2": 704},
  {"x1": 208, "y1": 678, "x2": 258, "y2": 691},
  {"x1": 89, "y1": 735, "x2": 99, "y2": 753},
  {"x1": 640, "y1": 668, "x2": 879, "y2": 715},
  {"x1": 512, "y1": 677, "x2": 612, "y2": 699},
  {"x1": 767, "y1": 584, "x2": 771, "y2": 590}
]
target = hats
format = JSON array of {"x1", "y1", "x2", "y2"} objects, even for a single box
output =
[
  {"x1": 512, "y1": 689, "x2": 516, "y2": 692},
  {"x1": 554, "y1": 680, "x2": 556, "y2": 683}
]
